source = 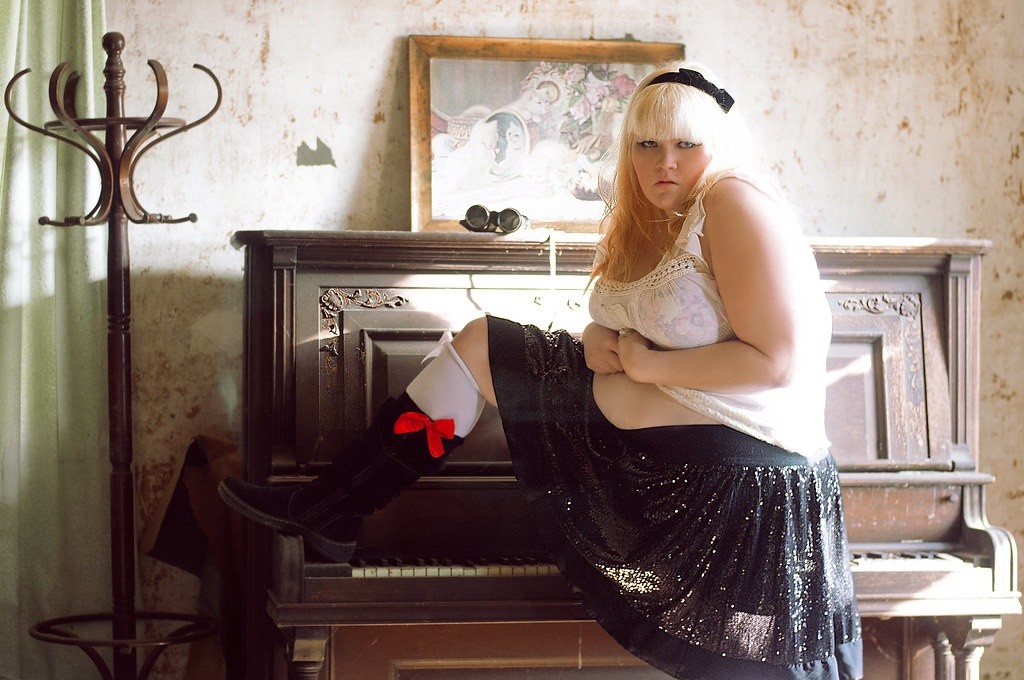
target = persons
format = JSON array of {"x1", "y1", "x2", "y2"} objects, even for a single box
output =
[{"x1": 217, "y1": 67, "x2": 864, "y2": 680}]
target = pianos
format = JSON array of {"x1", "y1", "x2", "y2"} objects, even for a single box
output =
[{"x1": 224, "y1": 239, "x2": 1022, "y2": 678}]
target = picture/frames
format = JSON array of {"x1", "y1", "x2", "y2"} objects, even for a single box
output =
[{"x1": 407, "y1": 35, "x2": 683, "y2": 232}]
